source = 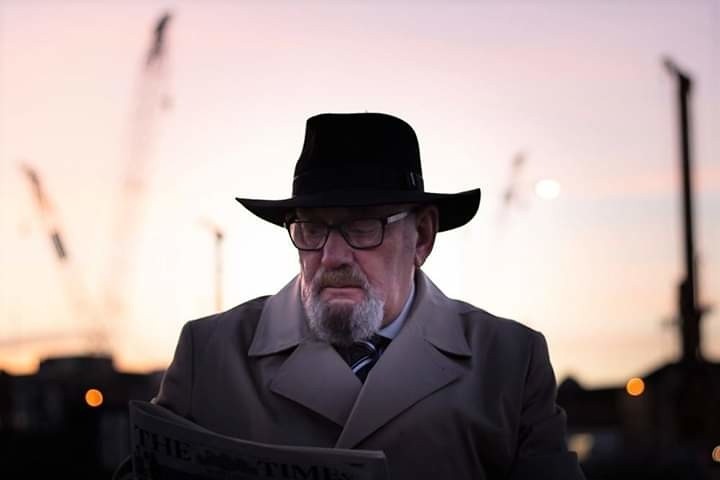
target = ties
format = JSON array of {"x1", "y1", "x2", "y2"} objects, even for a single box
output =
[{"x1": 347, "y1": 338, "x2": 380, "y2": 384}]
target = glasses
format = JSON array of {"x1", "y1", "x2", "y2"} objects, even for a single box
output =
[{"x1": 283, "y1": 208, "x2": 418, "y2": 251}]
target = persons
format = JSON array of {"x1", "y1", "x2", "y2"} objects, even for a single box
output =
[{"x1": 106, "y1": 112, "x2": 583, "y2": 480}]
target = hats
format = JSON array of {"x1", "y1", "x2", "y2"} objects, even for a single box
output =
[{"x1": 235, "y1": 113, "x2": 481, "y2": 232}]
